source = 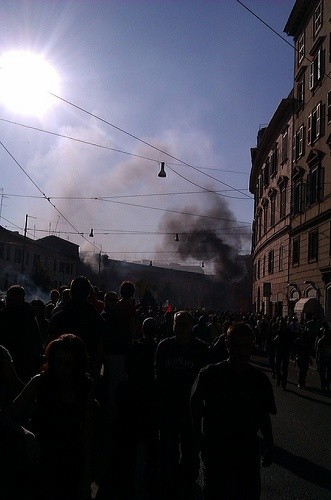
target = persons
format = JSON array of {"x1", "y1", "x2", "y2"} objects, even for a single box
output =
[
  {"x1": 190, "y1": 322, "x2": 280, "y2": 500},
  {"x1": 153, "y1": 309, "x2": 211, "y2": 463},
  {"x1": 4, "y1": 335, "x2": 108, "y2": 500},
  {"x1": 2, "y1": 271, "x2": 329, "y2": 403}
]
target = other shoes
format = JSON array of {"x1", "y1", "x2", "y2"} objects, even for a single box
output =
[
  {"x1": 320, "y1": 388, "x2": 331, "y2": 396},
  {"x1": 328, "y1": 454, "x2": 331, "y2": 463},
  {"x1": 310, "y1": 359, "x2": 313, "y2": 365},
  {"x1": 276, "y1": 378, "x2": 281, "y2": 387},
  {"x1": 270, "y1": 371, "x2": 274, "y2": 376},
  {"x1": 298, "y1": 382, "x2": 305, "y2": 390},
  {"x1": 292, "y1": 360, "x2": 296, "y2": 367}
]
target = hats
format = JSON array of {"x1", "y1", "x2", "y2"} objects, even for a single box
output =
[
  {"x1": 7, "y1": 286, "x2": 25, "y2": 297},
  {"x1": 174, "y1": 311, "x2": 193, "y2": 321}
]
